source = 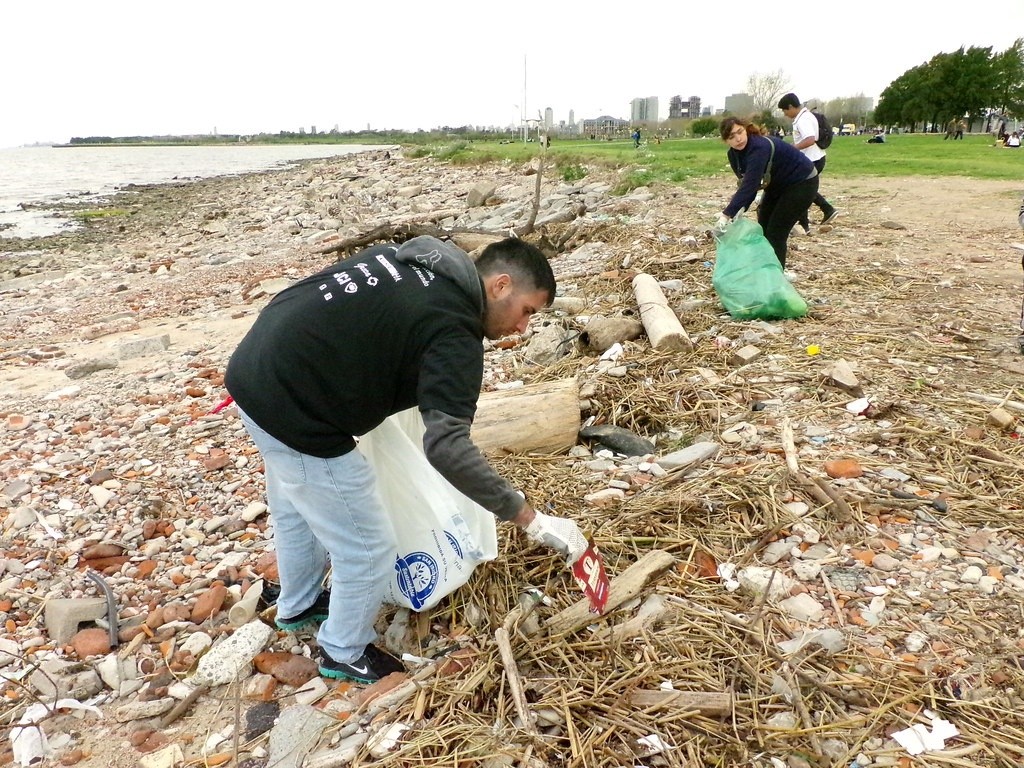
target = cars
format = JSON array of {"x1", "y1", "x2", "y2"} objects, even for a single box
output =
[{"x1": 926, "y1": 125, "x2": 940, "y2": 131}]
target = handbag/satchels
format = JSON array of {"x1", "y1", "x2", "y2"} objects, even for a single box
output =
[{"x1": 737, "y1": 173, "x2": 771, "y2": 189}]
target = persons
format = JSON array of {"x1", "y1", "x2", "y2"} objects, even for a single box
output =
[
  {"x1": 839, "y1": 123, "x2": 843, "y2": 134},
  {"x1": 778, "y1": 93, "x2": 839, "y2": 236},
  {"x1": 770, "y1": 124, "x2": 792, "y2": 140},
  {"x1": 223, "y1": 234, "x2": 589, "y2": 685},
  {"x1": 989, "y1": 122, "x2": 1024, "y2": 147},
  {"x1": 714, "y1": 117, "x2": 819, "y2": 271},
  {"x1": 1018, "y1": 198, "x2": 1024, "y2": 354},
  {"x1": 539, "y1": 134, "x2": 551, "y2": 147},
  {"x1": 860, "y1": 125, "x2": 885, "y2": 143},
  {"x1": 631, "y1": 128, "x2": 641, "y2": 147},
  {"x1": 944, "y1": 117, "x2": 965, "y2": 140}
]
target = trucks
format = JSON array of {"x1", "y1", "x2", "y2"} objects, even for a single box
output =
[{"x1": 842, "y1": 124, "x2": 855, "y2": 135}]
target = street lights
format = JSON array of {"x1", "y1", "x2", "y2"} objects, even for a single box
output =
[
  {"x1": 570, "y1": 127, "x2": 572, "y2": 140},
  {"x1": 516, "y1": 105, "x2": 522, "y2": 141}
]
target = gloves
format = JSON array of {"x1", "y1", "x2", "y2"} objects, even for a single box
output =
[
  {"x1": 521, "y1": 508, "x2": 588, "y2": 556},
  {"x1": 712, "y1": 217, "x2": 728, "y2": 234}
]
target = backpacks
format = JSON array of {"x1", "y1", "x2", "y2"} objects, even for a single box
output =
[{"x1": 796, "y1": 107, "x2": 833, "y2": 150}]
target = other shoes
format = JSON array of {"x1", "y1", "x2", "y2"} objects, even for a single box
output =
[
  {"x1": 820, "y1": 208, "x2": 838, "y2": 224},
  {"x1": 803, "y1": 228, "x2": 810, "y2": 234}
]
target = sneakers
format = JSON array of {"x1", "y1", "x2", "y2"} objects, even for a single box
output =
[
  {"x1": 274, "y1": 589, "x2": 330, "y2": 629},
  {"x1": 318, "y1": 644, "x2": 410, "y2": 685}
]
